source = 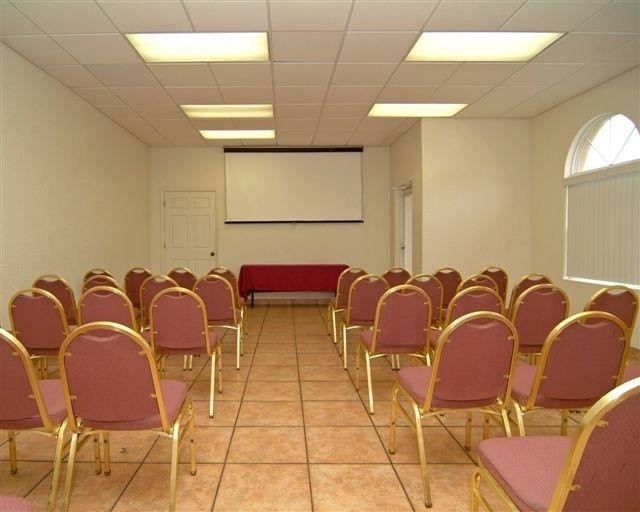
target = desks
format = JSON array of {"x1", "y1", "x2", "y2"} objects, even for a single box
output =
[{"x1": 238, "y1": 264, "x2": 351, "y2": 310}]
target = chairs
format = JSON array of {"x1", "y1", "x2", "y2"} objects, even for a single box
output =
[
  {"x1": 471, "y1": 377, "x2": 639, "y2": 511},
  {"x1": 8, "y1": 288, "x2": 72, "y2": 380},
  {"x1": 395, "y1": 273, "x2": 445, "y2": 370},
  {"x1": 389, "y1": 311, "x2": 519, "y2": 508},
  {"x1": 58, "y1": 321, "x2": 197, "y2": 512},
  {"x1": 582, "y1": 283, "x2": 639, "y2": 344},
  {"x1": 476, "y1": 266, "x2": 507, "y2": 308},
  {"x1": 149, "y1": 287, "x2": 222, "y2": 419},
  {"x1": 83, "y1": 267, "x2": 117, "y2": 296},
  {"x1": 510, "y1": 283, "x2": 570, "y2": 365},
  {"x1": 338, "y1": 274, "x2": 390, "y2": 369},
  {"x1": 383, "y1": 266, "x2": 411, "y2": 294},
  {"x1": 76, "y1": 286, "x2": 137, "y2": 337},
  {"x1": 444, "y1": 287, "x2": 504, "y2": 331},
  {"x1": 188, "y1": 274, "x2": 244, "y2": 371},
  {"x1": 206, "y1": 267, "x2": 247, "y2": 334},
  {"x1": 1, "y1": 328, "x2": 101, "y2": 476},
  {"x1": 619, "y1": 356, "x2": 639, "y2": 386},
  {"x1": 508, "y1": 272, "x2": 554, "y2": 324},
  {"x1": 124, "y1": 267, "x2": 157, "y2": 307},
  {"x1": 139, "y1": 274, "x2": 190, "y2": 371},
  {"x1": 434, "y1": 267, "x2": 463, "y2": 309},
  {"x1": 355, "y1": 285, "x2": 433, "y2": 416},
  {"x1": 327, "y1": 266, "x2": 370, "y2": 343},
  {"x1": 455, "y1": 275, "x2": 499, "y2": 294},
  {"x1": 31, "y1": 274, "x2": 79, "y2": 327},
  {"x1": 482, "y1": 311, "x2": 628, "y2": 439},
  {"x1": 1, "y1": 494, "x2": 41, "y2": 511},
  {"x1": 168, "y1": 267, "x2": 198, "y2": 295},
  {"x1": 83, "y1": 275, "x2": 123, "y2": 296}
]
